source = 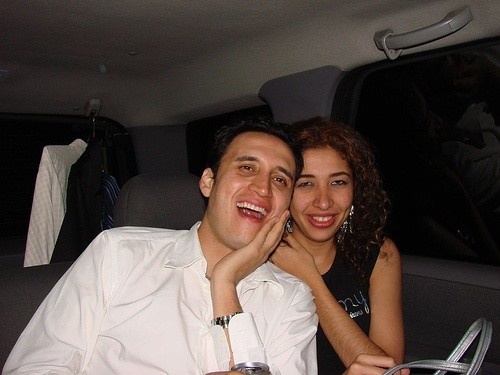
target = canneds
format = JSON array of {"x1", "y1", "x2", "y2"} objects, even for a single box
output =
[{"x1": 231, "y1": 361, "x2": 272, "y2": 375}]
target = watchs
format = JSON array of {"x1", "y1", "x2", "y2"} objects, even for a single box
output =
[{"x1": 210, "y1": 311, "x2": 246, "y2": 331}]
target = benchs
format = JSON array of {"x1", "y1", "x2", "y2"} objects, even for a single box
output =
[{"x1": 0, "y1": 171, "x2": 208, "y2": 374}]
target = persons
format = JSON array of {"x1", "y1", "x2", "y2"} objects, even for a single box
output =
[
  {"x1": 1, "y1": 114, "x2": 319, "y2": 375},
  {"x1": 270, "y1": 119, "x2": 412, "y2": 375}
]
